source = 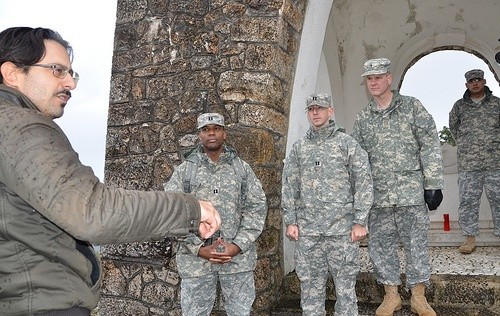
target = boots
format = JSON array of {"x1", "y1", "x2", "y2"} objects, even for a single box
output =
[
  {"x1": 376, "y1": 285, "x2": 402, "y2": 316},
  {"x1": 459, "y1": 235, "x2": 476, "y2": 253},
  {"x1": 410, "y1": 286, "x2": 437, "y2": 316}
]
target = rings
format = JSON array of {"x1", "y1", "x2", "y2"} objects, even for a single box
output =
[{"x1": 289, "y1": 237, "x2": 292, "y2": 240}]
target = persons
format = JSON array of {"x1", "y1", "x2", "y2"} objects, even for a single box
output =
[
  {"x1": 280, "y1": 92, "x2": 373, "y2": 316},
  {"x1": 162, "y1": 112, "x2": 268, "y2": 316},
  {"x1": 449, "y1": 69, "x2": 500, "y2": 253},
  {"x1": 0, "y1": 26, "x2": 221, "y2": 316},
  {"x1": 350, "y1": 58, "x2": 444, "y2": 316}
]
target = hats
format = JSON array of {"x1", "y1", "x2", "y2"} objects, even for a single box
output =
[
  {"x1": 305, "y1": 93, "x2": 331, "y2": 111},
  {"x1": 361, "y1": 58, "x2": 392, "y2": 77},
  {"x1": 197, "y1": 113, "x2": 224, "y2": 129},
  {"x1": 465, "y1": 69, "x2": 484, "y2": 82}
]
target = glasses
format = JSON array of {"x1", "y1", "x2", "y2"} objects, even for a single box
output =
[
  {"x1": 469, "y1": 78, "x2": 483, "y2": 83},
  {"x1": 11, "y1": 60, "x2": 79, "y2": 87}
]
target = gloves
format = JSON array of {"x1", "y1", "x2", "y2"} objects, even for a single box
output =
[{"x1": 424, "y1": 189, "x2": 444, "y2": 211}]
